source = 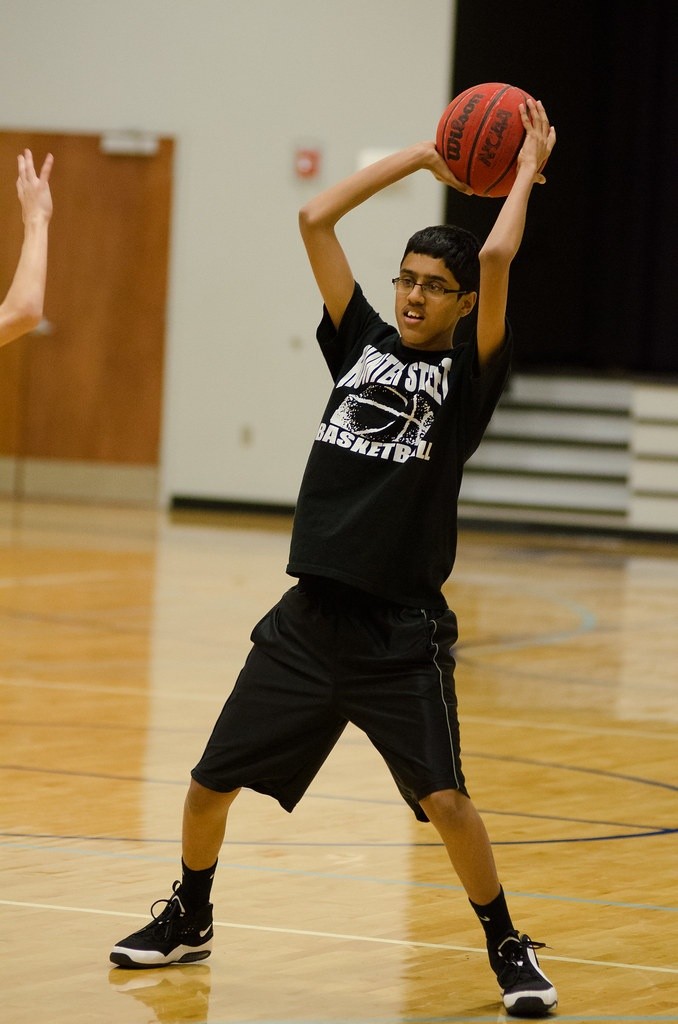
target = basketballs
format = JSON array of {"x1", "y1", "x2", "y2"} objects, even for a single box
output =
[{"x1": 434, "y1": 81, "x2": 550, "y2": 198}]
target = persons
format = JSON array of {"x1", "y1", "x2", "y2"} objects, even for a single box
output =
[
  {"x1": 0, "y1": 148, "x2": 55, "y2": 349},
  {"x1": 108, "y1": 100, "x2": 571, "y2": 1013}
]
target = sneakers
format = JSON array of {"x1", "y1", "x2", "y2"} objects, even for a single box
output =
[
  {"x1": 110, "y1": 881, "x2": 215, "y2": 969},
  {"x1": 490, "y1": 934, "x2": 557, "y2": 1016}
]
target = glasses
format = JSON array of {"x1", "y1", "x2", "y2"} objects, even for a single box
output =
[{"x1": 392, "y1": 277, "x2": 470, "y2": 299}]
